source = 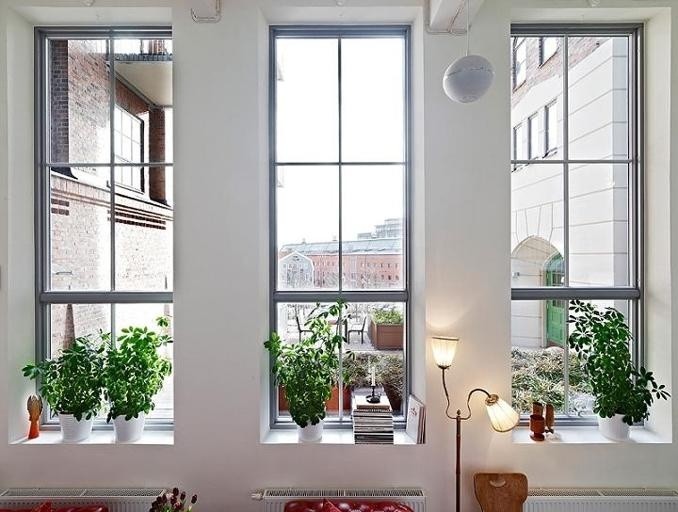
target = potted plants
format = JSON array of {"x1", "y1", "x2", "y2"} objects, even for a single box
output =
[
  {"x1": 564, "y1": 298, "x2": 673, "y2": 443},
  {"x1": 263, "y1": 295, "x2": 352, "y2": 441},
  {"x1": 21, "y1": 313, "x2": 175, "y2": 445},
  {"x1": 370, "y1": 305, "x2": 403, "y2": 350}
]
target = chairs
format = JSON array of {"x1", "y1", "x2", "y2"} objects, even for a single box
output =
[
  {"x1": 346, "y1": 314, "x2": 367, "y2": 345},
  {"x1": 296, "y1": 315, "x2": 317, "y2": 342}
]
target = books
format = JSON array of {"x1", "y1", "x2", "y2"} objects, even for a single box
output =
[
  {"x1": 351, "y1": 387, "x2": 395, "y2": 446},
  {"x1": 405, "y1": 392, "x2": 424, "y2": 443}
]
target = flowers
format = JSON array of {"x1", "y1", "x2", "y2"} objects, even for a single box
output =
[{"x1": 149, "y1": 484, "x2": 199, "y2": 512}]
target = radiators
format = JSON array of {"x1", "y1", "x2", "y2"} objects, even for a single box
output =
[
  {"x1": 2, "y1": 487, "x2": 167, "y2": 512},
  {"x1": 528, "y1": 484, "x2": 678, "y2": 512},
  {"x1": 249, "y1": 487, "x2": 428, "y2": 512}
]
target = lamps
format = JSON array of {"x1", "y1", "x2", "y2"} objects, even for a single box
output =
[
  {"x1": 442, "y1": 1, "x2": 496, "y2": 106},
  {"x1": 430, "y1": 334, "x2": 522, "y2": 511}
]
